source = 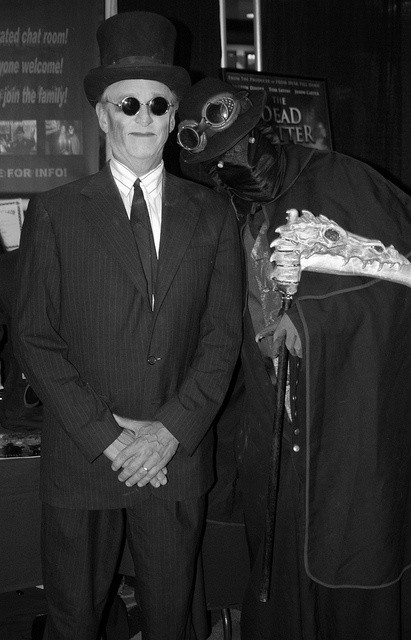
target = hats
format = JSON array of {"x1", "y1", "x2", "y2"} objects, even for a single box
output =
[
  {"x1": 171, "y1": 77, "x2": 268, "y2": 164},
  {"x1": 85, "y1": 10, "x2": 190, "y2": 105}
]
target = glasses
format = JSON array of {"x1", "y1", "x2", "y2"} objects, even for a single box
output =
[
  {"x1": 176, "y1": 89, "x2": 252, "y2": 155},
  {"x1": 105, "y1": 95, "x2": 176, "y2": 117}
]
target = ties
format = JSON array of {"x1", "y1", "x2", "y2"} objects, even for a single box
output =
[{"x1": 128, "y1": 177, "x2": 158, "y2": 308}]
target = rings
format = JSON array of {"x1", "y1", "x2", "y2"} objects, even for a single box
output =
[{"x1": 142, "y1": 465, "x2": 149, "y2": 471}]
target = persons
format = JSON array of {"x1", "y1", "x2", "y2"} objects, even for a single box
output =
[
  {"x1": 9, "y1": 9, "x2": 247, "y2": 639},
  {"x1": 174, "y1": 77, "x2": 410, "y2": 640}
]
